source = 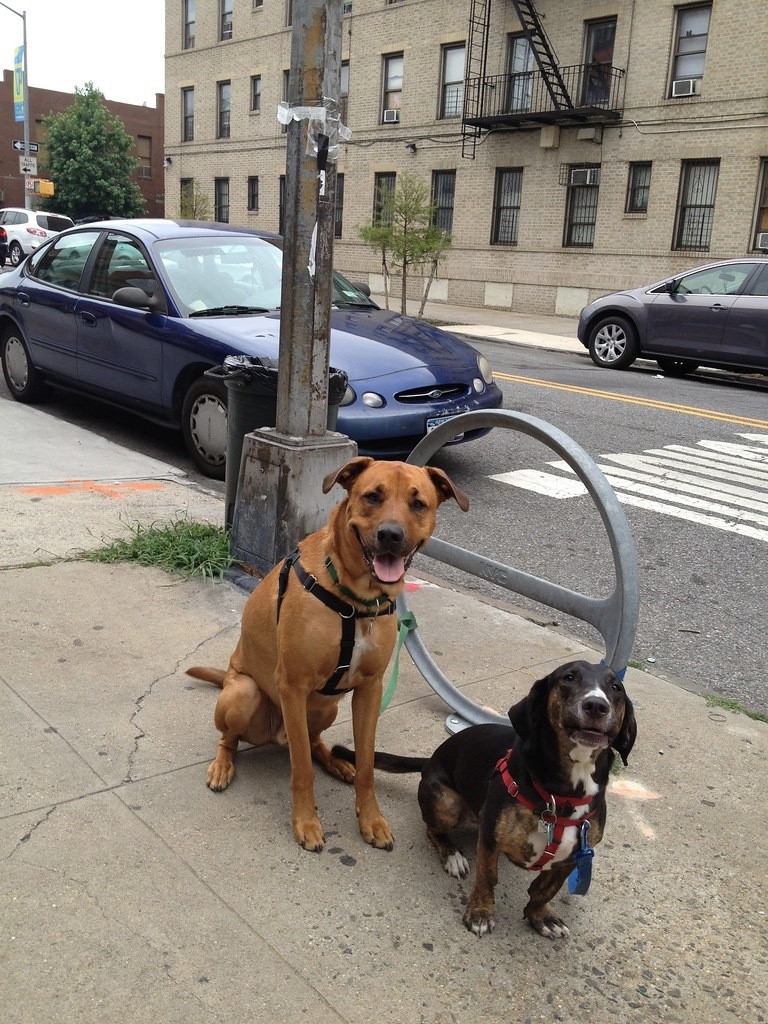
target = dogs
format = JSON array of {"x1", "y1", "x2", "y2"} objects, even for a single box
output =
[
  {"x1": 331, "y1": 660, "x2": 639, "y2": 940},
  {"x1": 187, "y1": 457, "x2": 470, "y2": 855}
]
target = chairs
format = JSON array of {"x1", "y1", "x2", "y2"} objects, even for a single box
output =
[
  {"x1": 109, "y1": 271, "x2": 156, "y2": 298},
  {"x1": 207, "y1": 270, "x2": 248, "y2": 303}
]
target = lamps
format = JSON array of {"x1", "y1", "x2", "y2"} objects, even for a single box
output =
[{"x1": 405, "y1": 143, "x2": 417, "y2": 153}]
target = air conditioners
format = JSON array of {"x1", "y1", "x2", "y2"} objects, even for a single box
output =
[
  {"x1": 672, "y1": 79, "x2": 697, "y2": 97},
  {"x1": 384, "y1": 109, "x2": 400, "y2": 123},
  {"x1": 138, "y1": 165, "x2": 151, "y2": 179},
  {"x1": 755, "y1": 232, "x2": 768, "y2": 250},
  {"x1": 223, "y1": 22, "x2": 233, "y2": 33},
  {"x1": 571, "y1": 168, "x2": 600, "y2": 186}
]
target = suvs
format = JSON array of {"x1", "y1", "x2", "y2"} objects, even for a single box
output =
[{"x1": 0, "y1": 207, "x2": 76, "y2": 266}]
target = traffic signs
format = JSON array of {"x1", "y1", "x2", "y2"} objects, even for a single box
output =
[
  {"x1": 12, "y1": 140, "x2": 39, "y2": 152},
  {"x1": 19, "y1": 156, "x2": 37, "y2": 174}
]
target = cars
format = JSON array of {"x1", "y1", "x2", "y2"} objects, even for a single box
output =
[
  {"x1": 198, "y1": 244, "x2": 256, "y2": 284},
  {"x1": 577, "y1": 259, "x2": 768, "y2": 375},
  {"x1": 75, "y1": 216, "x2": 126, "y2": 226},
  {"x1": 57, "y1": 234, "x2": 145, "y2": 260},
  {"x1": 0, "y1": 226, "x2": 8, "y2": 268},
  {"x1": 0, "y1": 219, "x2": 503, "y2": 481}
]
